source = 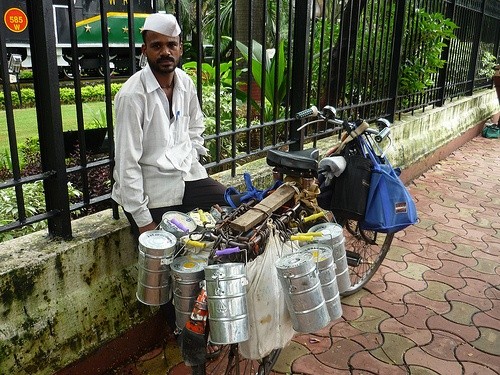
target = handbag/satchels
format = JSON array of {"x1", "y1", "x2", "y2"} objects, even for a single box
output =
[
  {"x1": 316, "y1": 120, "x2": 371, "y2": 222},
  {"x1": 237, "y1": 221, "x2": 301, "y2": 360},
  {"x1": 359, "y1": 135, "x2": 418, "y2": 234},
  {"x1": 482, "y1": 122, "x2": 500, "y2": 139}
]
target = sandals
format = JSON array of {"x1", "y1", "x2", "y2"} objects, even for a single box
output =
[{"x1": 206, "y1": 342, "x2": 221, "y2": 358}]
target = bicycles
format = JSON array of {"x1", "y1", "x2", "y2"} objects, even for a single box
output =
[{"x1": 184, "y1": 106, "x2": 396, "y2": 375}]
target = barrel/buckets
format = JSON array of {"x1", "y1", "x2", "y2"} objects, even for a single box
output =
[
  {"x1": 135, "y1": 205, "x2": 251, "y2": 345},
  {"x1": 275, "y1": 235, "x2": 331, "y2": 333},
  {"x1": 300, "y1": 212, "x2": 351, "y2": 294},
  {"x1": 290, "y1": 231, "x2": 343, "y2": 321}
]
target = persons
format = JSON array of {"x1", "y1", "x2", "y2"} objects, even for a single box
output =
[{"x1": 112, "y1": 13, "x2": 241, "y2": 357}]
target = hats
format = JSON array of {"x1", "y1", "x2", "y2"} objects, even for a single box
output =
[{"x1": 140, "y1": 13, "x2": 181, "y2": 38}]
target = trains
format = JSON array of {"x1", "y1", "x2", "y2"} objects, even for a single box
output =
[{"x1": 0, "y1": 0, "x2": 216, "y2": 83}]
